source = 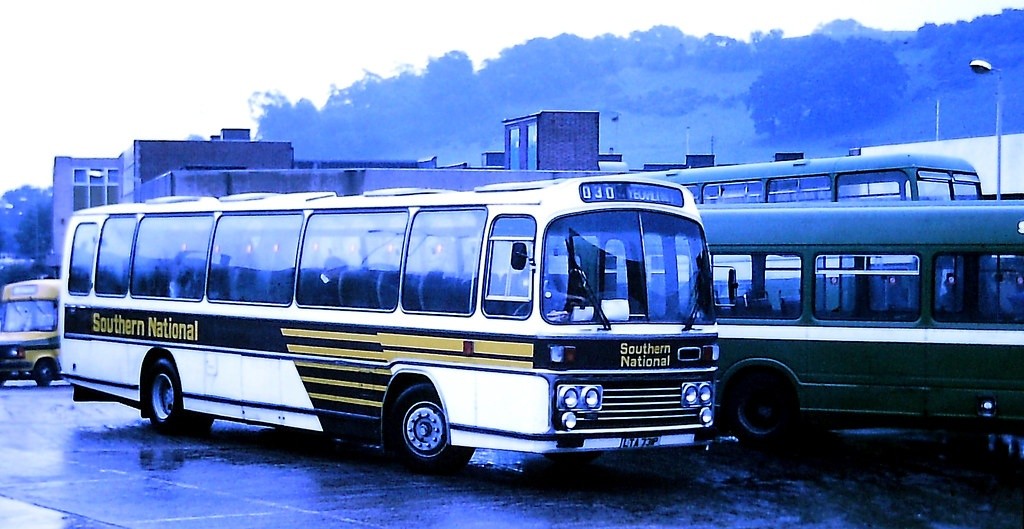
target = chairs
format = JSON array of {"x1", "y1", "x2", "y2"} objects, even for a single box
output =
[
  {"x1": 68, "y1": 251, "x2": 575, "y2": 320},
  {"x1": 712, "y1": 269, "x2": 1024, "y2": 330}
]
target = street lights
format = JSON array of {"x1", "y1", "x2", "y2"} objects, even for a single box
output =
[
  {"x1": 969, "y1": 58, "x2": 1002, "y2": 200},
  {"x1": 89, "y1": 171, "x2": 143, "y2": 205}
]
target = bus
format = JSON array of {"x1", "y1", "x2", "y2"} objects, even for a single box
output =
[
  {"x1": 60, "y1": 174, "x2": 721, "y2": 472},
  {"x1": 676, "y1": 200, "x2": 1023, "y2": 450},
  {"x1": 0, "y1": 279, "x2": 66, "y2": 386},
  {"x1": 616, "y1": 152, "x2": 984, "y2": 314}
]
